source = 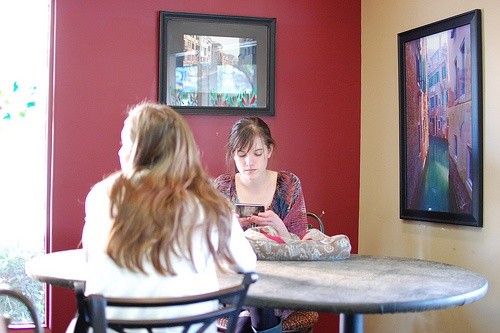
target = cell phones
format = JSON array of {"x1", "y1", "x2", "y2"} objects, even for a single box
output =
[{"x1": 235, "y1": 203, "x2": 265, "y2": 217}]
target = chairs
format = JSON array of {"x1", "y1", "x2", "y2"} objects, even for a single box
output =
[
  {"x1": 87, "y1": 271, "x2": 258, "y2": 333},
  {"x1": 216, "y1": 211, "x2": 323, "y2": 333}
]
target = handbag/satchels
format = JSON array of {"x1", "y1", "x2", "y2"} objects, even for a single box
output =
[{"x1": 242, "y1": 226, "x2": 352, "y2": 261}]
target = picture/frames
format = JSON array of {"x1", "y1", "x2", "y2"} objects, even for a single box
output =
[
  {"x1": 397, "y1": 9, "x2": 484, "y2": 227},
  {"x1": 157, "y1": 11, "x2": 276, "y2": 117}
]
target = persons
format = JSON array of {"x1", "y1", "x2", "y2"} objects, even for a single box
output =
[
  {"x1": 211, "y1": 115, "x2": 309, "y2": 333},
  {"x1": 64, "y1": 97, "x2": 258, "y2": 333}
]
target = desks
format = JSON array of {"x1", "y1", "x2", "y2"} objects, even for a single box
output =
[{"x1": 25, "y1": 248, "x2": 488, "y2": 333}]
